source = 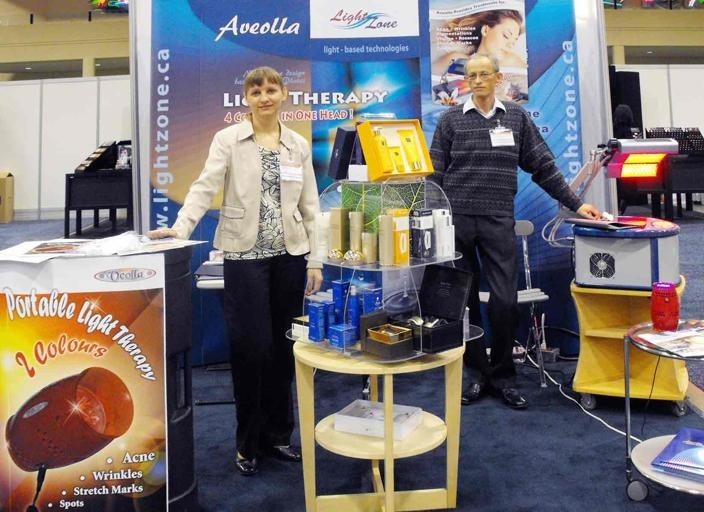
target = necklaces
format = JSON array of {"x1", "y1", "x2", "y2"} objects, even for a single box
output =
[{"x1": 473, "y1": 96, "x2": 492, "y2": 114}]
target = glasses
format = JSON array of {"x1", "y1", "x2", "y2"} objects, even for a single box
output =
[{"x1": 463, "y1": 71, "x2": 495, "y2": 81}]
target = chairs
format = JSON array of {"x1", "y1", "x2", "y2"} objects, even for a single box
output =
[{"x1": 479, "y1": 220, "x2": 550, "y2": 392}]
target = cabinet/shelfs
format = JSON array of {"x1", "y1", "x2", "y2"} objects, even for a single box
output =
[
  {"x1": 285, "y1": 179, "x2": 487, "y2": 365},
  {"x1": 570, "y1": 272, "x2": 687, "y2": 418}
]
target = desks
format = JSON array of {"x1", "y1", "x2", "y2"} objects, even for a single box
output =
[
  {"x1": 63, "y1": 169, "x2": 133, "y2": 238},
  {"x1": 291, "y1": 340, "x2": 466, "y2": 512}
]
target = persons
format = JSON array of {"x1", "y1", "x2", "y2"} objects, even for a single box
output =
[
  {"x1": 118, "y1": 146, "x2": 132, "y2": 166},
  {"x1": 427, "y1": 51, "x2": 606, "y2": 410},
  {"x1": 432, "y1": 9, "x2": 526, "y2": 78},
  {"x1": 508, "y1": 87, "x2": 521, "y2": 100},
  {"x1": 146, "y1": 63, "x2": 328, "y2": 477}
]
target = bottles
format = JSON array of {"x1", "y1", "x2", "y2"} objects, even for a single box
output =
[{"x1": 650, "y1": 282, "x2": 680, "y2": 332}]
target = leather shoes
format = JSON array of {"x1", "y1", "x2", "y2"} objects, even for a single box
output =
[
  {"x1": 235, "y1": 451, "x2": 259, "y2": 476},
  {"x1": 268, "y1": 444, "x2": 302, "y2": 462},
  {"x1": 461, "y1": 383, "x2": 485, "y2": 405},
  {"x1": 491, "y1": 387, "x2": 529, "y2": 410}
]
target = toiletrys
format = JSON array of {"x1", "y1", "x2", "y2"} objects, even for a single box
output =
[
  {"x1": 348, "y1": 211, "x2": 364, "y2": 261},
  {"x1": 346, "y1": 285, "x2": 361, "y2": 341},
  {"x1": 377, "y1": 214, "x2": 394, "y2": 266},
  {"x1": 361, "y1": 232, "x2": 377, "y2": 264}
]
